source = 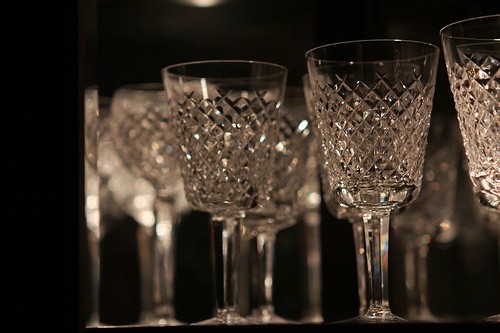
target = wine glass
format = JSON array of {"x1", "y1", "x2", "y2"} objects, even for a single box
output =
[
  {"x1": 306, "y1": 39, "x2": 439, "y2": 323},
  {"x1": 162, "y1": 60, "x2": 287, "y2": 325},
  {"x1": 441, "y1": 15, "x2": 500, "y2": 323},
  {"x1": 112, "y1": 88, "x2": 193, "y2": 327}
]
